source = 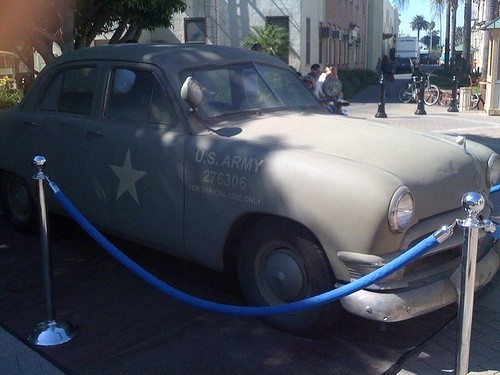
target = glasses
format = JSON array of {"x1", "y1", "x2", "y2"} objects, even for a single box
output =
[{"x1": 304, "y1": 80, "x2": 312, "y2": 83}]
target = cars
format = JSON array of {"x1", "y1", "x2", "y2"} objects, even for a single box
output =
[{"x1": 0, "y1": 41, "x2": 500, "y2": 339}]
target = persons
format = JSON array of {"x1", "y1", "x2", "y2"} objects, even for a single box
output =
[
  {"x1": 251, "y1": 43, "x2": 263, "y2": 52},
  {"x1": 289, "y1": 55, "x2": 393, "y2": 116}
]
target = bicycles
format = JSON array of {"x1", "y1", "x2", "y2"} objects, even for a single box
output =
[
  {"x1": 437, "y1": 74, "x2": 480, "y2": 110},
  {"x1": 398, "y1": 72, "x2": 440, "y2": 106}
]
